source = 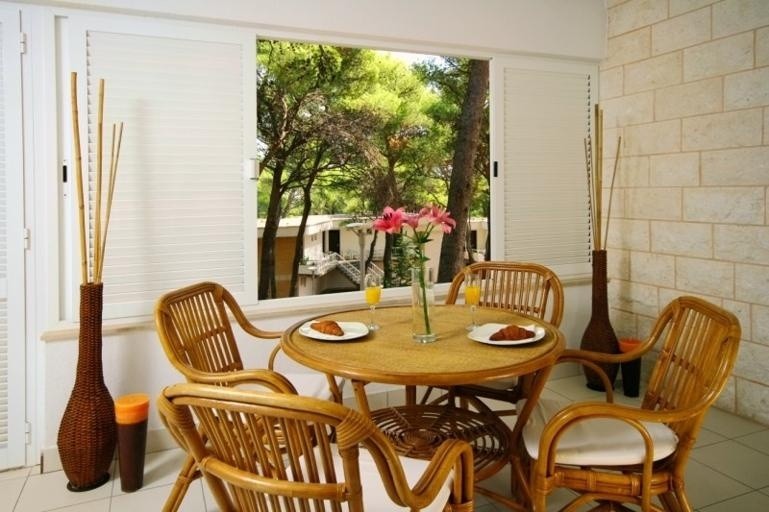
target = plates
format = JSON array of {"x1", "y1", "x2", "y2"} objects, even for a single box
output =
[
  {"x1": 297, "y1": 320, "x2": 368, "y2": 340},
  {"x1": 466, "y1": 322, "x2": 548, "y2": 346}
]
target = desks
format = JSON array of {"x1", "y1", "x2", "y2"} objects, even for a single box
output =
[{"x1": 282, "y1": 307, "x2": 564, "y2": 510}]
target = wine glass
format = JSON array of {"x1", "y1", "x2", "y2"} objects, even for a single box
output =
[
  {"x1": 459, "y1": 272, "x2": 483, "y2": 331},
  {"x1": 363, "y1": 274, "x2": 385, "y2": 331}
]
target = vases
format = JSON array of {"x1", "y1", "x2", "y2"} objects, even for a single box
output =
[
  {"x1": 580, "y1": 250, "x2": 621, "y2": 392},
  {"x1": 57, "y1": 284, "x2": 118, "y2": 491},
  {"x1": 408, "y1": 269, "x2": 438, "y2": 343}
]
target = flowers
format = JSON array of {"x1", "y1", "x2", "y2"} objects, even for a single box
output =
[{"x1": 372, "y1": 204, "x2": 457, "y2": 333}]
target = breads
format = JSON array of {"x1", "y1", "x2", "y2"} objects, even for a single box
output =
[
  {"x1": 310, "y1": 319, "x2": 344, "y2": 337},
  {"x1": 489, "y1": 325, "x2": 536, "y2": 341}
]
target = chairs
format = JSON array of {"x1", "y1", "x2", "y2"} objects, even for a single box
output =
[
  {"x1": 151, "y1": 280, "x2": 341, "y2": 510},
  {"x1": 416, "y1": 259, "x2": 564, "y2": 510},
  {"x1": 517, "y1": 294, "x2": 743, "y2": 510},
  {"x1": 151, "y1": 382, "x2": 474, "y2": 512}
]
